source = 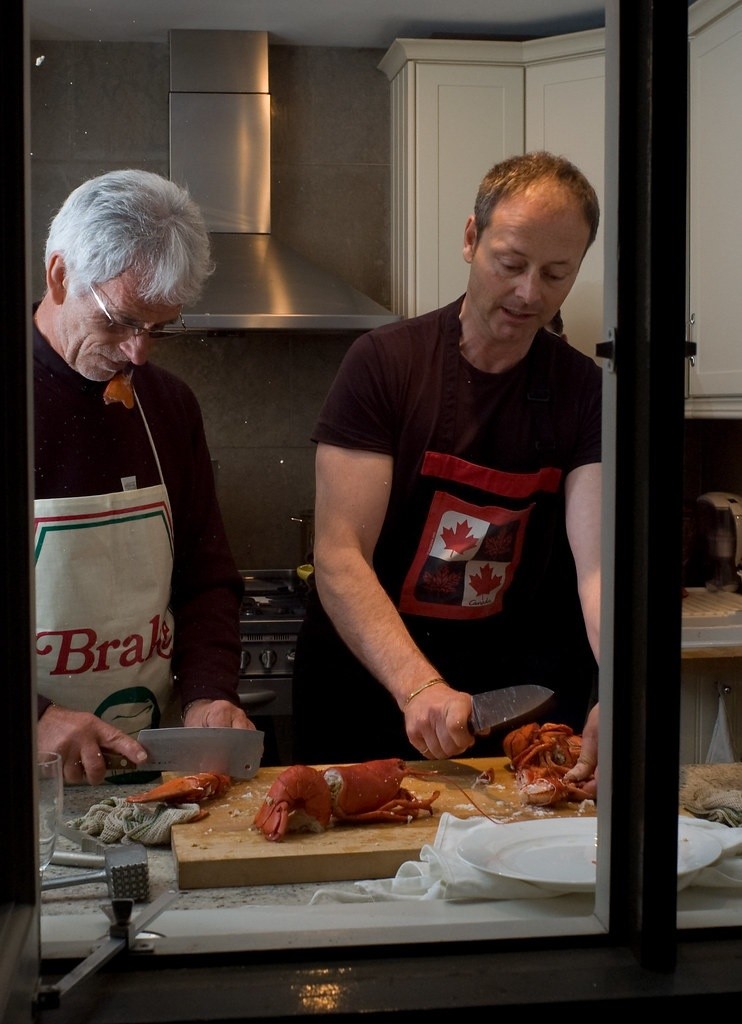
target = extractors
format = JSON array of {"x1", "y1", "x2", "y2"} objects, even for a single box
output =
[{"x1": 83, "y1": 30, "x2": 402, "y2": 333}]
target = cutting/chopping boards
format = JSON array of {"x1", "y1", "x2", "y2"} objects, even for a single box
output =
[{"x1": 162, "y1": 756, "x2": 598, "y2": 889}]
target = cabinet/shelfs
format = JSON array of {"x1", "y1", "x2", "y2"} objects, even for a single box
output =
[
  {"x1": 680, "y1": 657, "x2": 742, "y2": 769},
  {"x1": 684, "y1": 1, "x2": 742, "y2": 424},
  {"x1": 376, "y1": 27, "x2": 607, "y2": 375}
]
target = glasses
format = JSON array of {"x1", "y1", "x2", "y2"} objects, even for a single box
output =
[{"x1": 89, "y1": 283, "x2": 188, "y2": 341}]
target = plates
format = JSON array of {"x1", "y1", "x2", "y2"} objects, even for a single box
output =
[{"x1": 457, "y1": 817, "x2": 724, "y2": 890}]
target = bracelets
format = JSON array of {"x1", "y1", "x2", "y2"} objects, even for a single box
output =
[
  {"x1": 180, "y1": 698, "x2": 211, "y2": 726},
  {"x1": 401, "y1": 678, "x2": 447, "y2": 713}
]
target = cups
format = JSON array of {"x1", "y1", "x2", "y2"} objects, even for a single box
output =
[{"x1": 33, "y1": 751, "x2": 65, "y2": 873}]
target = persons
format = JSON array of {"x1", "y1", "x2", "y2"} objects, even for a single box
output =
[
  {"x1": 294, "y1": 149, "x2": 606, "y2": 798},
  {"x1": 30, "y1": 169, "x2": 262, "y2": 790}
]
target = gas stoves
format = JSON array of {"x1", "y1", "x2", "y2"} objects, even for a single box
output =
[{"x1": 228, "y1": 575, "x2": 304, "y2": 677}]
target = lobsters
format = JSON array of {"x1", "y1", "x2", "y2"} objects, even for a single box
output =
[
  {"x1": 503, "y1": 722, "x2": 582, "y2": 806},
  {"x1": 255, "y1": 758, "x2": 439, "y2": 843},
  {"x1": 127, "y1": 772, "x2": 232, "y2": 806}
]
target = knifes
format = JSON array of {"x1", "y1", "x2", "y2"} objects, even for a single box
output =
[
  {"x1": 466, "y1": 684, "x2": 554, "y2": 735},
  {"x1": 94, "y1": 727, "x2": 265, "y2": 780}
]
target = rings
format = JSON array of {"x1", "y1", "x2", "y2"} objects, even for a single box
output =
[{"x1": 420, "y1": 748, "x2": 429, "y2": 754}]
